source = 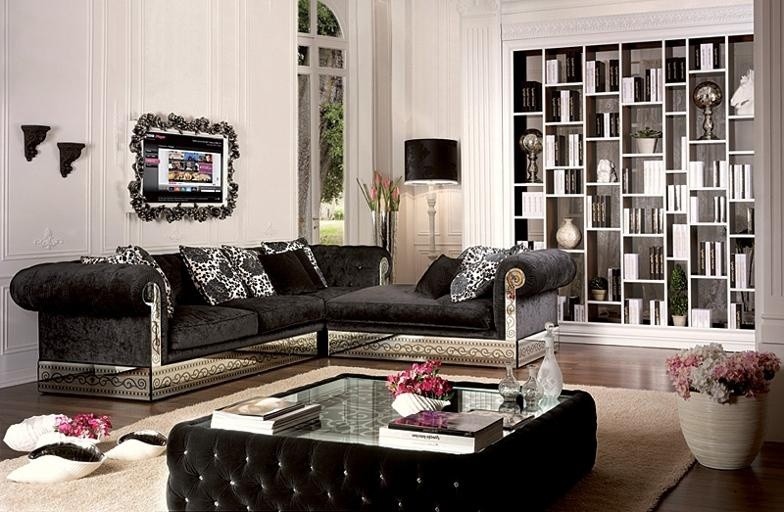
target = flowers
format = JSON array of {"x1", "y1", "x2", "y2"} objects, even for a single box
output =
[
  {"x1": 55, "y1": 411, "x2": 113, "y2": 441},
  {"x1": 666, "y1": 344, "x2": 782, "y2": 403},
  {"x1": 386, "y1": 359, "x2": 457, "y2": 401},
  {"x1": 357, "y1": 171, "x2": 403, "y2": 261}
]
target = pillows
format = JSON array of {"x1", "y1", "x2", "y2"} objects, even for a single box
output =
[
  {"x1": 81, "y1": 236, "x2": 328, "y2": 317},
  {"x1": 415, "y1": 243, "x2": 531, "y2": 303}
]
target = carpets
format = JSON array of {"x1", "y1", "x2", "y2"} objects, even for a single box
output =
[{"x1": 1, "y1": 364, "x2": 695, "y2": 512}]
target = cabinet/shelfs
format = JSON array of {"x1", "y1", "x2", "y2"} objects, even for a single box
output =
[{"x1": 501, "y1": 22, "x2": 754, "y2": 353}]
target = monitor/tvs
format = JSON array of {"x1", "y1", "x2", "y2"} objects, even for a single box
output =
[{"x1": 129, "y1": 121, "x2": 229, "y2": 208}]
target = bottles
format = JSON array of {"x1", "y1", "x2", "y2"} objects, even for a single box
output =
[
  {"x1": 536, "y1": 322, "x2": 563, "y2": 399},
  {"x1": 519, "y1": 364, "x2": 543, "y2": 402},
  {"x1": 556, "y1": 218, "x2": 581, "y2": 250}
]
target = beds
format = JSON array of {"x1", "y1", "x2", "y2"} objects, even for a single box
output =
[{"x1": 324, "y1": 248, "x2": 577, "y2": 369}]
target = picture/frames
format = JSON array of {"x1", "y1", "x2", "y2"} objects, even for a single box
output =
[{"x1": 128, "y1": 113, "x2": 240, "y2": 223}]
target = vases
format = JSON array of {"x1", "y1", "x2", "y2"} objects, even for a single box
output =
[
  {"x1": 370, "y1": 211, "x2": 398, "y2": 284},
  {"x1": 522, "y1": 365, "x2": 543, "y2": 411},
  {"x1": 678, "y1": 390, "x2": 768, "y2": 469}
]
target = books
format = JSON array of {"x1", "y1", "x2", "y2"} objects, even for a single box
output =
[
  {"x1": 623, "y1": 161, "x2": 664, "y2": 194},
  {"x1": 376, "y1": 401, "x2": 504, "y2": 453},
  {"x1": 546, "y1": 51, "x2": 583, "y2": 194},
  {"x1": 729, "y1": 164, "x2": 753, "y2": 199},
  {"x1": 519, "y1": 81, "x2": 542, "y2": 112},
  {"x1": 623, "y1": 207, "x2": 664, "y2": 326},
  {"x1": 730, "y1": 254, "x2": 749, "y2": 330},
  {"x1": 680, "y1": 137, "x2": 687, "y2": 170},
  {"x1": 556, "y1": 295, "x2": 585, "y2": 322},
  {"x1": 608, "y1": 267, "x2": 621, "y2": 302},
  {"x1": 587, "y1": 195, "x2": 611, "y2": 228},
  {"x1": 666, "y1": 56, "x2": 686, "y2": 83},
  {"x1": 585, "y1": 59, "x2": 620, "y2": 93},
  {"x1": 212, "y1": 399, "x2": 321, "y2": 437},
  {"x1": 689, "y1": 160, "x2": 726, "y2": 275},
  {"x1": 596, "y1": 112, "x2": 619, "y2": 137},
  {"x1": 622, "y1": 68, "x2": 662, "y2": 104},
  {"x1": 694, "y1": 44, "x2": 720, "y2": 70},
  {"x1": 667, "y1": 184, "x2": 687, "y2": 212}
]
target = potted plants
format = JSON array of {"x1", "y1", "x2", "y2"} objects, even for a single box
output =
[
  {"x1": 630, "y1": 127, "x2": 662, "y2": 154},
  {"x1": 669, "y1": 263, "x2": 688, "y2": 326},
  {"x1": 590, "y1": 276, "x2": 607, "y2": 301}
]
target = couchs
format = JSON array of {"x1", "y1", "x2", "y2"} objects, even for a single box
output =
[{"x1": 9, "y1": 244, "x2": 393, "y2": 403}]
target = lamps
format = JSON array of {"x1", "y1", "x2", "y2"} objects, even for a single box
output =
[{"x1": 404, "y1": 138, "x2": 458, "y2": 269}]
